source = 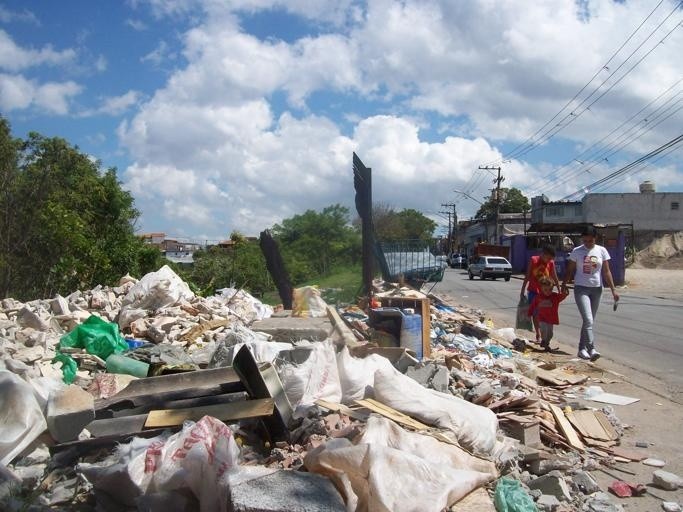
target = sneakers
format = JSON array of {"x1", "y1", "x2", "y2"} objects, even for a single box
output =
[{"x1": 577, "y1": 348, "x2": 601, "y2": 362}]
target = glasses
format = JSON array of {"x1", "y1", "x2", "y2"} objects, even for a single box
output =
[{"x1": 613, "y1": 295, "x2": 619, "y2": 311}]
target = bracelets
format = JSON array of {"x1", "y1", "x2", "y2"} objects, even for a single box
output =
[
  {"x1": 610, "y1": 288, "x2": 616, "y2": 290},
  {"x1": 562, "y1": 282, "x2": 567, "y2": 285}
]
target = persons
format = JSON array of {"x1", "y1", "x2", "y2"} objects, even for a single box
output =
[
  {"x1": 527, "y1": 276, "x2": 569, "y2": 353},
  {"x1": 520, "y1": 244, "x2": 560, "y2": 343},
  {"x1": 463, "y1": 256, "x2": 467, "y2": 268},
  {"x1": 457, "y1": 254, "x2": 463, "y2": 269},
  {"x1": 561, "y1": 228, "x2": 620, "y2": 362}
]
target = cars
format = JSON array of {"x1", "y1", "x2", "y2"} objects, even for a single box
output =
[
  {"x1": 450, "y1": 253, "x2": 467, "y2": 268},
  {"x1": 467, "y1": 256, "x2": 513, "y2": 281}
]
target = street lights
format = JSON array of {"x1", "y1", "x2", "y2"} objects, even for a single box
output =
[{"x1": 453, "y1": 189, "x2": 500, "y2": 246}]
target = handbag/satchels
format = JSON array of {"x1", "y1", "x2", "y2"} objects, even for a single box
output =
[{"x1": 516, "y1": 293, "x2": 532, "y2": 332}]
